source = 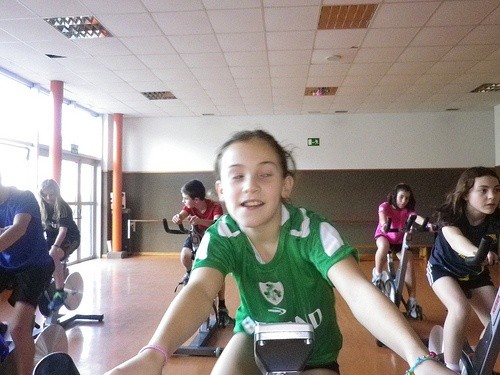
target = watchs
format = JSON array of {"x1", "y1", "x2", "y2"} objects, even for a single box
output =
[{"x1": 52, "y1": 244, "x2": 61, "y2": 252}]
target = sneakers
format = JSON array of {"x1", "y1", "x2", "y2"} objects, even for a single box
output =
[
  {"x1": 369, "y1": 268, "x2": 384, "y2": 291},
  {"x1": 216, "y1": 308, "x2": 230, "y2": 327},
  {"x1": 182, "y1": 270, "x2": 191, "y2": 286},
  {"x1": 405, "y1": 296, "x2": 421, "y2": 318},
  {"x1": 49, "y1": 288, "x2": 68, "y2": 309}
]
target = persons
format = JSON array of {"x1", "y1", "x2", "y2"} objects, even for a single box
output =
[
  {"x1": 0, "y1": 172, "x2": 56, "y2": 375},
  {"x1": 426, "y1": 166, "x2": 500, "y2": 375},
  {"x1": 40, "y1": 178, "x2": 80, "y2": 311},
  {"x1": 370, "y1": 183, "x2": 437, "y2": 320},
  {"x1": 171, "y1": 179, "x2": 232, "y2": 327},
  {"x1": 103, "y1": 128, "x2": 459, "y2": 375},
  {"x1": 311, "y1": 87, "x2": 331, "y2": 96}
]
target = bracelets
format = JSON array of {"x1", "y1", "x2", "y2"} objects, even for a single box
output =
[
  {"x1": 139, "y1": 344, "x2": 169, "y2": 367},
  {"x1": 382, "y1": 222, "x2": 386, "y2": 226},
  {"x1": 405, "y1": 351, "x2": 447, "y2": 375}
]
